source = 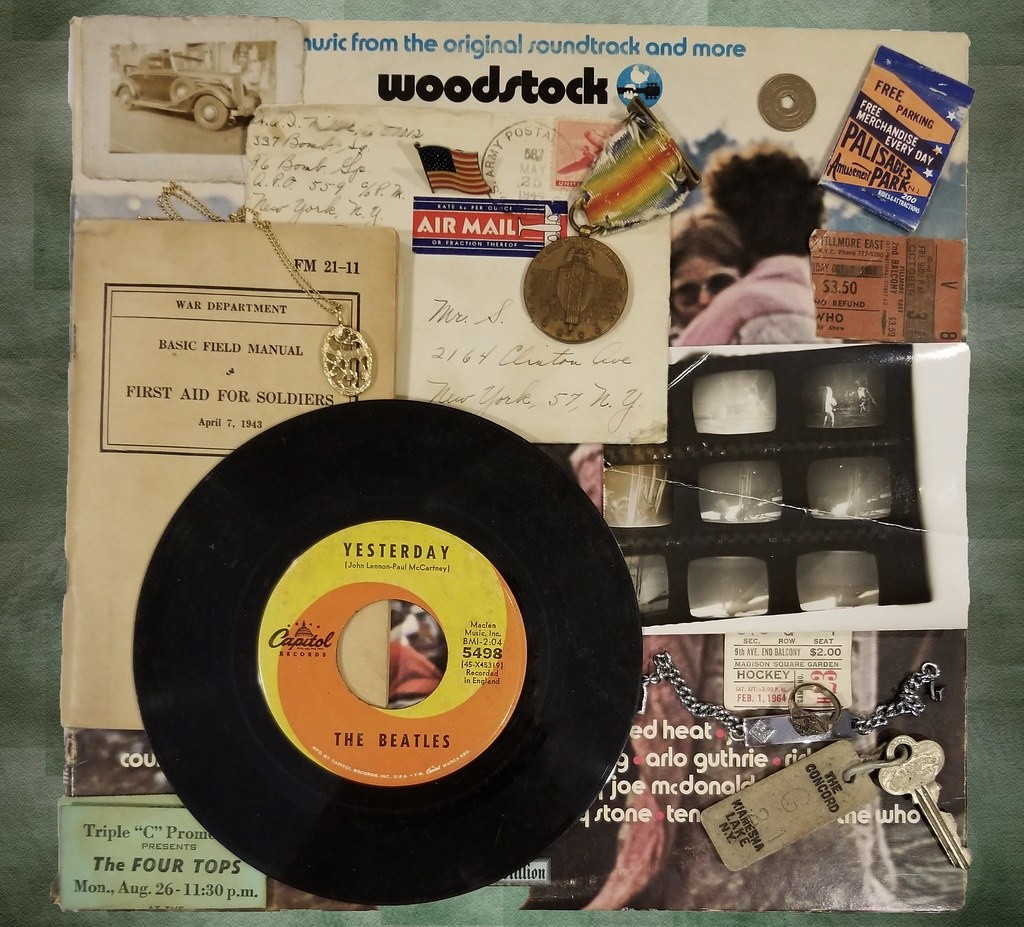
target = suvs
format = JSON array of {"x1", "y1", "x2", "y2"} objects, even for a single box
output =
[{"x1": 114, "y1": 48, "x2": 264, "y2": 132}]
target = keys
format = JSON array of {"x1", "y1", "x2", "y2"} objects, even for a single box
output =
[{"x1": 879, "y1": 736, "x2": 974, "y2": 869}]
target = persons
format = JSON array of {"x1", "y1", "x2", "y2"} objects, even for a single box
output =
[{"x1": 669, "y1": 139, "x2": 839, "y2": 343}]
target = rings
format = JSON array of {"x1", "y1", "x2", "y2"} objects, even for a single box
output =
[{"x1": 789, "y1": 682, "x2": 841, "y2": 737}]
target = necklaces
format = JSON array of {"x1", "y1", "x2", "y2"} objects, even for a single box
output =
[{"x1": 137, "y1": 180, "x2": 373, "y2": 396}]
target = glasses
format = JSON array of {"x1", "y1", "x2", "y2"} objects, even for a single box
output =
[{"x1": 672, "y1": 272, "x2": 739, "y2": 305}]
